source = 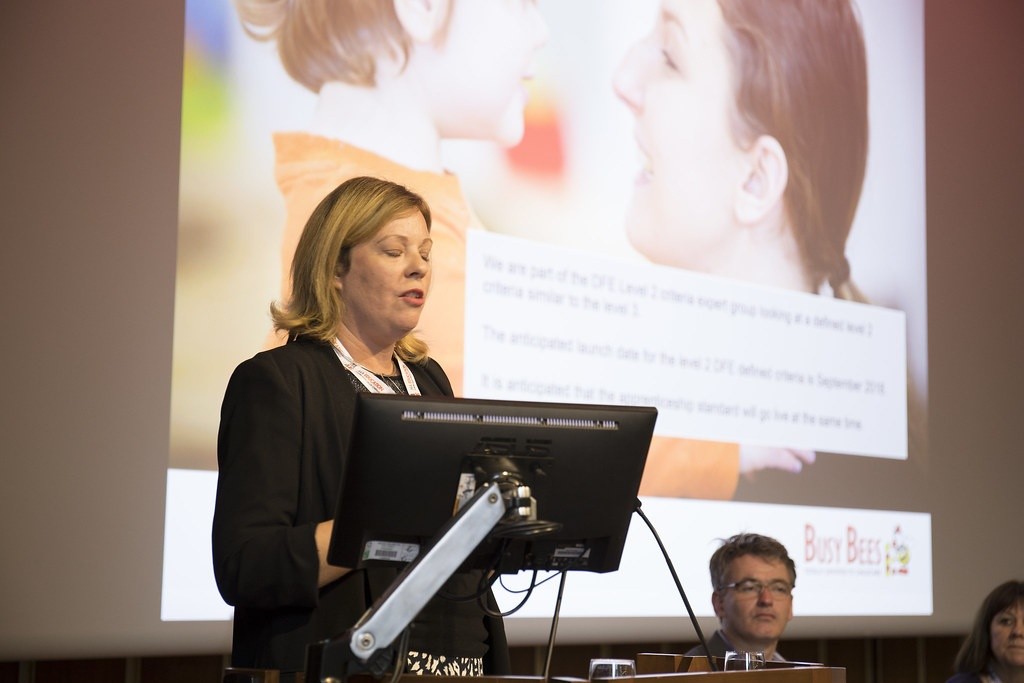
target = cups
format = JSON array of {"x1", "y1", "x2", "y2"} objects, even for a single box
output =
[
  {"x1": 589, "y1": 659, "x2": 636, "y2": 682},
  {"x1": 724, "y1": 650, "x2": 766, "y2": 671}
]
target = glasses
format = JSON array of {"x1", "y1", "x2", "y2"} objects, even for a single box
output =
[{"x1": 721, "y1": 576, "x2": 793, "y2": 602}]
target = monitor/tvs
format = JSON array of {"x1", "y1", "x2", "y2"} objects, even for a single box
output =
[{"x1": 326, "y1": 392, "x2": 658, "y2": 574}]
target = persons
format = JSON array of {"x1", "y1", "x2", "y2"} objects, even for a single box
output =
[
  {"x1": 211, "y1": 176, "x2": 512, "y2": 683},
  {"x1": 942, "y1": 578, "x2": 1024, "y2": 683},
  {"x1": 682, "y1": 532, "x2": 796, "y2": 661},
  {"x1": 613, "y1": 0, "x2": 933, "y2": 514},
  {"x1": 228, "y1": 0, "x2": 817, "y2": 502}
]
